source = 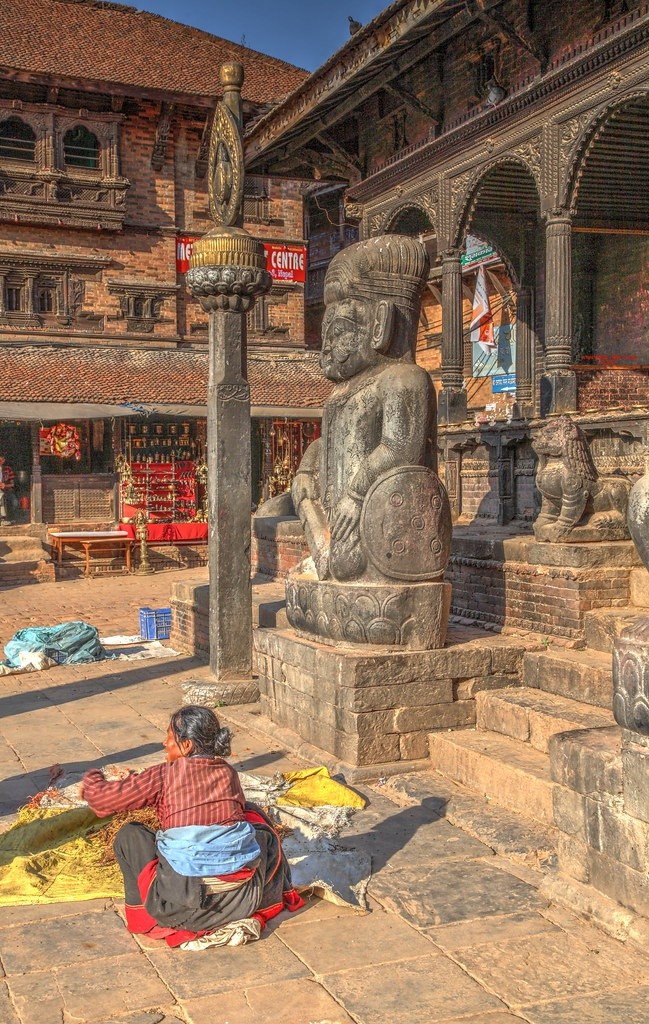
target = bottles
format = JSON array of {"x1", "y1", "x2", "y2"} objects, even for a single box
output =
[{"x1": 136, "y1": 438, "x2": 190, "y2": 463}]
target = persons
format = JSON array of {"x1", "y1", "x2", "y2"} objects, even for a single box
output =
[
  {"x1": 292, "y1": 234, "x2": 436, "y2": 583},
  {"x1": 0, "y1": 453, "x2": 18, "y2": 526},
  {"x1": 82, "y1": 706, "x2": 291, "y2": 929}
]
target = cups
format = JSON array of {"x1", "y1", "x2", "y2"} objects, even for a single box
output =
[
  {"x1": 155, "y1": 426, "x2": 162, "y2": 434},
  {"x1": 141, "y1": 426, "x2": 148, "y2": 434},
  {"x1": 182, "y1": 424, "x2": 189, "y2": 434},
  {"x1": 129, "y1": 426, "x2": 135, "y2": 434},
  {"x1": 170, "y1": 426, "x2": 177, "y2": 434}
]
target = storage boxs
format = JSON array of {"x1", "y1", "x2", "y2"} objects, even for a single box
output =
[{"x1": 139, "y1": 607, "x2": 171, "y2": 640}]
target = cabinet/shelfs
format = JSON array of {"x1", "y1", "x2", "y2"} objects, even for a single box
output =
[{"x1": 117, "y1": 423, "x2": 208, "y2": 545}]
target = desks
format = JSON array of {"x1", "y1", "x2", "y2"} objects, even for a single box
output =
[
  {"x1": 81, "y1": 538, "x2": 135, "y2": 578},
  {"x1": 50, "y1": 530, "x2": 128, "y2": 567}
]
